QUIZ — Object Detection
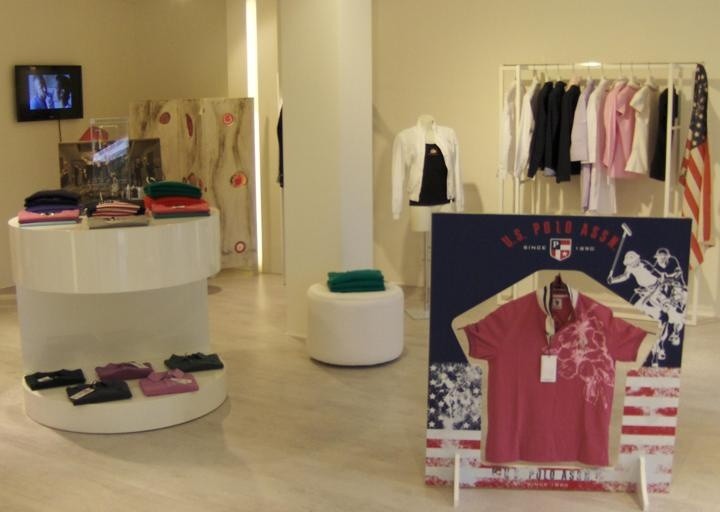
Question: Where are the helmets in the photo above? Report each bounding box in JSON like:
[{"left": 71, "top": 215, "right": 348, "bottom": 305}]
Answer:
[{"left": 623, "top": 251, "right": 640, "bottom": 266}]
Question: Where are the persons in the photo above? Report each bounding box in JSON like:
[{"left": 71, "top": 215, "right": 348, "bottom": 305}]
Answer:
[
  {"left": 648, "top": 248, "right": 686, "bottom": 347},
  {"left": 390, "top": 115, "right": 464, "bottom": 232},
  {"left": 29, "top": 76, "right": 52, "bottom": 109},
  {"left": 54, "top": 76, "right": 70, "bottom": 108},
  {"left": 608, "top": 251, "right": 670, "bottom": 363}
]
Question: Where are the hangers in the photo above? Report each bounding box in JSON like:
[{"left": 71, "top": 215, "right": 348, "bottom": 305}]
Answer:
[{"left": 547, "top": 62, "right": 663, "bottom": 94}]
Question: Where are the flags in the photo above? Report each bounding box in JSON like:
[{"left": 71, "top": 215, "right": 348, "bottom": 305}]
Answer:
[{"left": 679, "top": 65, "right": 713, "bottom": 269}]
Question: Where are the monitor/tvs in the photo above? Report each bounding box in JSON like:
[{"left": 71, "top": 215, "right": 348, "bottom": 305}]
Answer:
[{"left": 14, "top": 64, "right": 84, "bottom": 123}]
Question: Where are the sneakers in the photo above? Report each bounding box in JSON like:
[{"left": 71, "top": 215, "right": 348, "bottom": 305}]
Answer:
[{"left": 652, "top": 331, "right": 680, "bottom": 360}]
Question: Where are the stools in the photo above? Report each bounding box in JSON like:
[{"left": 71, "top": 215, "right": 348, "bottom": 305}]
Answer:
[{"left": 304, "top": 282, "right": 405, "bottom": 367}]
